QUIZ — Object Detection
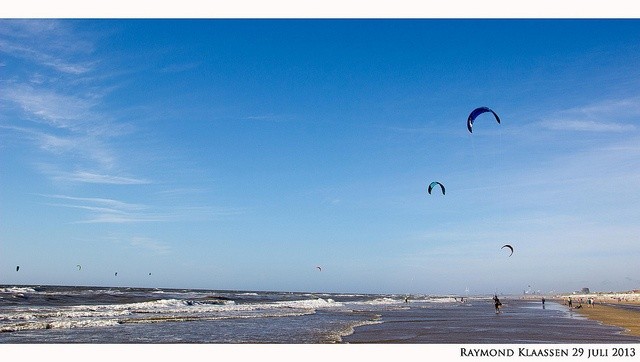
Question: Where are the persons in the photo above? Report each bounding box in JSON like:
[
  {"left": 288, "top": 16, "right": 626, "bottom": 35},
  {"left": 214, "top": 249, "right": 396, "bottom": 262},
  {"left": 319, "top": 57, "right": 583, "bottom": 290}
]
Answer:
[
  {"left": 493, "top": 296, "right": 500, "bottom": 314},
  {"left": 542, "top": 298, "right": 545, "bottom": 305},
  {"left": 567, "top": 297, "right": 595, "bottom": 309}
]
